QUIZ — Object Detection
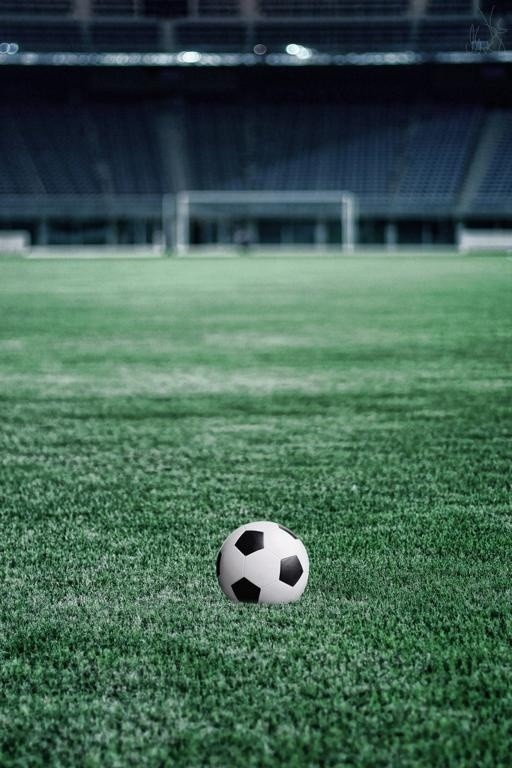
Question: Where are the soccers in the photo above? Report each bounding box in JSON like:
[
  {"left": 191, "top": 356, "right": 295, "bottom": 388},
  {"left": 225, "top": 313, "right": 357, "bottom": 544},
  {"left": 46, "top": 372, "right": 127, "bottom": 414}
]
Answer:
[{"left": 216, "top": 521, "right": 310, "bottom": 603}]
